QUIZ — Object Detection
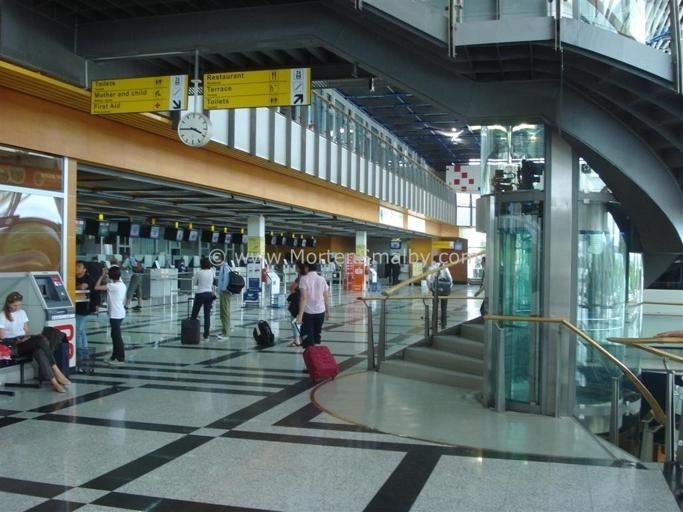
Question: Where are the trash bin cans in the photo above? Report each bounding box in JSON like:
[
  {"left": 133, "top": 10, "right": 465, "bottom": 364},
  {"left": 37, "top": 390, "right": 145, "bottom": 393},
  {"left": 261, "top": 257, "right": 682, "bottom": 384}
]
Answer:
[{"left": 76, "top": 347, "right": 96, "bottom": 375}]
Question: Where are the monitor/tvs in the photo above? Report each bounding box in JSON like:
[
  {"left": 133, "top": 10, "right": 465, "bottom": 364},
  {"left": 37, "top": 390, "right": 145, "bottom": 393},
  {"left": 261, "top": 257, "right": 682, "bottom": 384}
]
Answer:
[
  {"left": 87, "top": 221, "right": 110, "bottom": 238},
  {"left": 183, "top": 230, "right": 199, "bottom": 241},
  {"left": 141, "top": 225, "right": 159, "bottom": 239},
  {"left": 74, "top": 220, "right": 85, "bottom": 235},
  {"left": 118, "top": 222, "right": 140, "bottom": 238},
  {"left": 164, "top": 229, "right": 184, "bottom": 240},
  {"left": 37, "top": 284, "right": 47, "bottom": 297},
  {"left": 201, "top": 230, "right": 317, "bottom": 249}
]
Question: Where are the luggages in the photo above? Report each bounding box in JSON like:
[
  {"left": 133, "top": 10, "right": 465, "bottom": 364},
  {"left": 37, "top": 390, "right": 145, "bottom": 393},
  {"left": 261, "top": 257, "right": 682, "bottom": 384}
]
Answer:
[
  {"left": 181, "top": 298, "right": 200, "bottom": 344},
  {"left": 39, "top": 327, "right": 69, "bottom": 378},
  {"left": 295, "top": 322, "right": 338, "bottom": 379}
]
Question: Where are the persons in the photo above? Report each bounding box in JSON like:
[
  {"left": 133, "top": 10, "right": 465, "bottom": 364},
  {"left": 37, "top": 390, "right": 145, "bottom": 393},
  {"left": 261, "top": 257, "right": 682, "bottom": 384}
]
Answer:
[
  {"left": 191, "top": 257, "right": 215, "bottom": 342},
  {"left": 215, "top": 251, "right": 233, "bottom": 341},
  {"left": 474, "top": 278, "right": 488, "bottom": 314},
  {"left": 180, "top": 253, "right": 400, "bottom": 293},
  {"left": 473, "top": 257, "right": 486, "bottom": 315},
  {"left": 287, "top": 260, "right": 303, "bottom": 348},
  {"left": 74, "top": 259, "right": 93, "bottom": 374},
  {"left": 654, "top": 329, "right": 683, "bottom": 338},
  {"left": 427, "top": 255, "right": 453, "bottom": 330},
  {"left": 490, "top": 157, "right": 536, "bottom": 229},
  {"left": 116, "top": 253, "right": 144, "bottom": 311},
  {"left": 296, "top": 260, "right": 331, "bottom": 373},
  {"left": 93, "top": 265, "right": 129, "bottom": 364},
  {"left": 0, "top": 291, "right": 72, "bottom": 393}
]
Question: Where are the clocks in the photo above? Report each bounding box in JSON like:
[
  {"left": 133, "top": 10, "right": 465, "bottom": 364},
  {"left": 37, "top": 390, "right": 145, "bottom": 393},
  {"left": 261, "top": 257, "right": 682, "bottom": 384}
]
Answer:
[{"left": 177, "top": 112, "right": 212, "bottom": 147}]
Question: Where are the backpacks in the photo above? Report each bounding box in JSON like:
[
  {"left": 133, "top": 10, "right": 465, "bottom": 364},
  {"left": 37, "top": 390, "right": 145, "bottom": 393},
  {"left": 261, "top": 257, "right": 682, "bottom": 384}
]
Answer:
[
  {"left": 223, "top": 265, "right": 245, "bottom": 294},
  {"left": 253, "top": 320, "right": 274, "bottom": 345}
]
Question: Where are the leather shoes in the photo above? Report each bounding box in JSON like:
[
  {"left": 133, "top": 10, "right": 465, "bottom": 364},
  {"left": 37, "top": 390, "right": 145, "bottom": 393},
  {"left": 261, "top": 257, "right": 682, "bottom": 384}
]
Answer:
[{"left": 58, "top": 379, "right": 71, "bottom": 392}]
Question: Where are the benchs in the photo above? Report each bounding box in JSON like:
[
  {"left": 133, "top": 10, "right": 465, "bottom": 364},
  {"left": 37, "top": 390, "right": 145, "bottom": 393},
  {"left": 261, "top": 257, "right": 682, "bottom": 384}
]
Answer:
[{"left": 0, "top": 355, "right": 40, "bottom": 395}]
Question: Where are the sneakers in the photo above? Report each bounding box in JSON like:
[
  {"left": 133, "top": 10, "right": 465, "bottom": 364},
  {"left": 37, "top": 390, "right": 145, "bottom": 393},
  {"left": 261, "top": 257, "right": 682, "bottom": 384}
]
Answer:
[
  {"left": 104, "top": 358, "right": 124, "bottom": 364},
  {"left": 124, "top": 304, "right": 143, "bottom": 309}
]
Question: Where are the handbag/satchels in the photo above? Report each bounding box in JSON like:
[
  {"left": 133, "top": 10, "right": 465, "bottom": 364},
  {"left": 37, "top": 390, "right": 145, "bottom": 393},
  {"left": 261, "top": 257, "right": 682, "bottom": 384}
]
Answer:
[
  {"left": 132, "top": 261, "right": 144, "bottom": 273},
  {"left": 433, "top": 278, "right": 451, "bottom": 296}
]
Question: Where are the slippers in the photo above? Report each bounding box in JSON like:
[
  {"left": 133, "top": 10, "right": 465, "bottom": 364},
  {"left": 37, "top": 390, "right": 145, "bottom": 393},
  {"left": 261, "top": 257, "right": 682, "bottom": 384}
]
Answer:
[{"left": 288, "top": 342, "right": 301, "bottom": 346}]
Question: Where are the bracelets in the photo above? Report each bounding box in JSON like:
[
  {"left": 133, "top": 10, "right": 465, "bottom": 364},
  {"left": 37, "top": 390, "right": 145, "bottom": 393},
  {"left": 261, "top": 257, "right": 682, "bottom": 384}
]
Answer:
[{"left": 679, "top": 329, "right": 682, "bottom": 338}]
